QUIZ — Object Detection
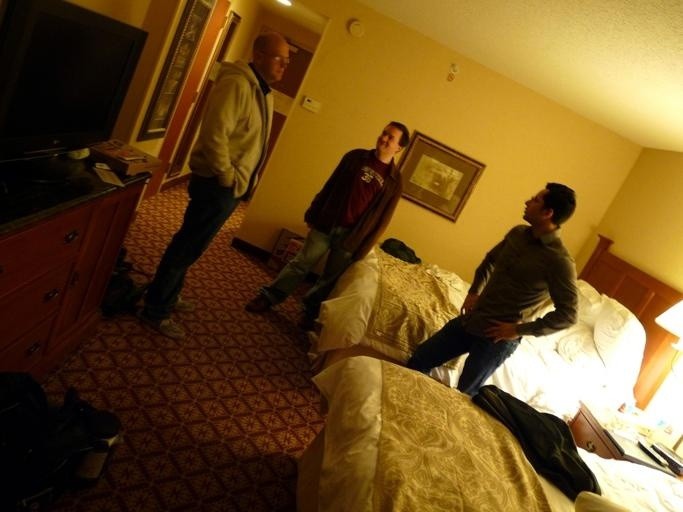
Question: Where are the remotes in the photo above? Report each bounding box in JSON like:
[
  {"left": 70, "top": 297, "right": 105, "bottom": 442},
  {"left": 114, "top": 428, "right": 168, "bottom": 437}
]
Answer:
[{"left": 639, "top": 438, "right": 669, "bottom": 467}]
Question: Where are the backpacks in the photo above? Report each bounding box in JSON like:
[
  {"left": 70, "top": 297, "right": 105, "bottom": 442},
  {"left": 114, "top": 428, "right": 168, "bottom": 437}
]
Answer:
[{"left": 0, "top": 370, "right": 82, "bottom": 512}]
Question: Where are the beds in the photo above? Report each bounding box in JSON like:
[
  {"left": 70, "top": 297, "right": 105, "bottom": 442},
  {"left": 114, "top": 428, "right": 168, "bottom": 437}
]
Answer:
[
  {"left": 294, "top": 356, "right": 683, "bottom": 512},
  {"left": 307, "top": 231, "right": 683, "bottom": 411}
]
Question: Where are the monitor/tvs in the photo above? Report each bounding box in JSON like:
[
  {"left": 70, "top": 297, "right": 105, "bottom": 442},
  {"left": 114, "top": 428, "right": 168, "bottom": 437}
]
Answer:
[{"left": 0, "top": 0, "right": 149, "bottom": 195}]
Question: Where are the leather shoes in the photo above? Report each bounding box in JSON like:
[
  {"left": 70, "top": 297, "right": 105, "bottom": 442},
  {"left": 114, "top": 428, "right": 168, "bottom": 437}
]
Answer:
[
  {"left": 245, "top": 294, "right": 271, "bottom": 312},
  {"left": 301, "top": 310, "right": 313, "bottom": 329}
]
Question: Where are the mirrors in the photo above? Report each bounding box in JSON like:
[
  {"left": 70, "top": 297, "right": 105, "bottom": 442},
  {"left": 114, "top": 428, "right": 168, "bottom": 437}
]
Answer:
[{"left": 167, "top": 10, "right": 242, "bottom": 178}]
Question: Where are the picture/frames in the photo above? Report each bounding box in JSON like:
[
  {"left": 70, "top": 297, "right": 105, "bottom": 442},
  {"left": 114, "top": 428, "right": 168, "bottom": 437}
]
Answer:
[
  {"left": 135, "top": 0, "right": 220, "bottom": 143},
  {"left": 398, "top": 131, "right": 485, "bottom": 223}
]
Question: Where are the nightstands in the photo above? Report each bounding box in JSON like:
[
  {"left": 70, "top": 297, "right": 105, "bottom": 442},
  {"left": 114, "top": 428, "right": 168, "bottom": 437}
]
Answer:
[{"left": 567, "top": 400, "right": 682, "bottom": 482}]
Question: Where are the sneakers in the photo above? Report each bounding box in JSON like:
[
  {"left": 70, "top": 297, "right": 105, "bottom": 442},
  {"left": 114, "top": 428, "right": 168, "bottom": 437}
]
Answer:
[
  {"left": 136, "top": 308, "right": 186, "bottom": 340},
  {"left": 141, "top": 287, "right": 196, "bottom": 312}
]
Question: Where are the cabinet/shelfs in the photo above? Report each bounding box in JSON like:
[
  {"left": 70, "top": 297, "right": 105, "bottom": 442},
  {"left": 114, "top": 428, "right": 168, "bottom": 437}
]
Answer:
[{"left": 1, "top": 177, "right": 147, "bottom": 386}]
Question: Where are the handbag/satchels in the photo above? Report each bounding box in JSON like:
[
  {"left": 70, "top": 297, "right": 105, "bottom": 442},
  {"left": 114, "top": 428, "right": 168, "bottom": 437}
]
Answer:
[
  {"left": 380, "top": 238, "right": 422, "bottom": 264},
  {"left": 53, "top": 385, "right": 123, "bottom": 493},
  {"left": 99, "top": 244, "right": 155, "bottom": 317}
]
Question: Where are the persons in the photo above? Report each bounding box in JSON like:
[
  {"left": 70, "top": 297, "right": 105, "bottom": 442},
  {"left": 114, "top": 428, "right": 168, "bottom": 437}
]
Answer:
[
  {"left": 134, "top": 29, "right": 291, "bottom": 341},
  {"left": 243, "top": 119, "right": 411, "bottom": 334},
  {"left": 400, "top": 181, "right": 582, "bottom": 397}
]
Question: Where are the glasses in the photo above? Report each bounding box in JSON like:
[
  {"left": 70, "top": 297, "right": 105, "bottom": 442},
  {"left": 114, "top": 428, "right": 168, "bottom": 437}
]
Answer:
[{"left": 259, "top": 49, "right": 291, "bottom": 65}]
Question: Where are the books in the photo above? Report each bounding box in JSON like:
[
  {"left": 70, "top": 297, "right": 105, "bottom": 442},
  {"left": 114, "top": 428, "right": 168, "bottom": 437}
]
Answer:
[{"left": 603, "top": 427, "right": 683, "bottom": 478}]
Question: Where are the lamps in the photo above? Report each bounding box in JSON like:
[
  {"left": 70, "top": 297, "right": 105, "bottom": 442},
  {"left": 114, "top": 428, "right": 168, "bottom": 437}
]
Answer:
[{"left": 651, "top": 297, "right": 683, "bottom": 474}]
